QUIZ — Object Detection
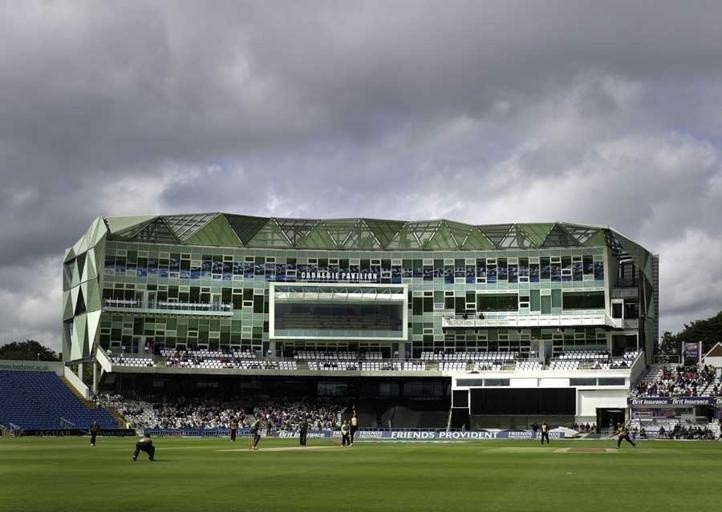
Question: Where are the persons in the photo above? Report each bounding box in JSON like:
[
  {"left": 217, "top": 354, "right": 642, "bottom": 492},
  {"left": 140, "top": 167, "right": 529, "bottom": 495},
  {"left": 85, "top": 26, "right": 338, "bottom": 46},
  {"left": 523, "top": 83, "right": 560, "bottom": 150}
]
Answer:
[
  {"left": 533, "top": 365, "right": 721, "bottom": 448},
  {"left": 91, "top": 392, "right": 361, "bottom": 462},
  {"left": 346, "top": 364, "right": 397, "bottom": 371}
]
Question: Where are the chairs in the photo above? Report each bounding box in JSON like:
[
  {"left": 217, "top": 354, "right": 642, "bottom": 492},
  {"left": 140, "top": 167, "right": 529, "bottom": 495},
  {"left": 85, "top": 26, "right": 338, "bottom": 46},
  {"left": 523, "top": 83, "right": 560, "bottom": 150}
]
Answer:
[{"left": 108, "top": 348, "right": 638, "bottom": 372}]
